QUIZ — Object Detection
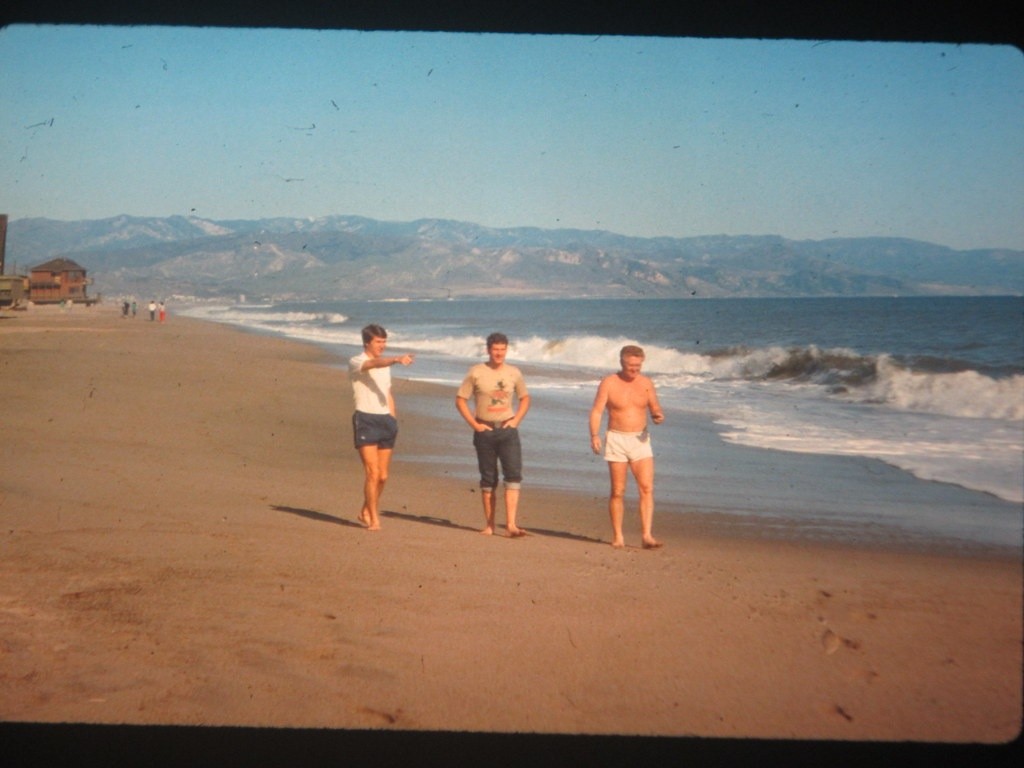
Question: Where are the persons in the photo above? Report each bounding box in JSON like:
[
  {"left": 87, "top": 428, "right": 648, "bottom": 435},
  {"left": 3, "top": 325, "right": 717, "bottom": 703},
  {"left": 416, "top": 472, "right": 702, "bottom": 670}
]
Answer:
[
  {"left": 122, "top": 300, "right": 166, "bottom": 323},
  {"left": 589, "top": 344, "right": 666, "bottom": 549},
  {"left": 348, "top": 323, "right": 415, "bottom": 530},
  {"left": 455, "top": 332, "right": 531, "bottom": 538},
  {"left": 59, "top": 297, "right": 73, "bottom": 312}
]
